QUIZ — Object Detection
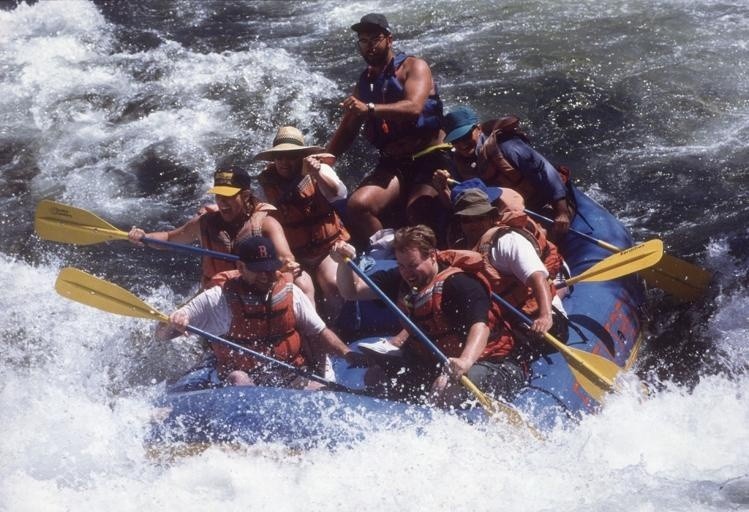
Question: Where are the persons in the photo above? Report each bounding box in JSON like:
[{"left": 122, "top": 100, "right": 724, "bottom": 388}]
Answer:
[
  {"left": 130, "top": 106, "right": 568, "bottom": 408},
  {"left": 317, "top": 13, "right": 456, "bottom": 236}
]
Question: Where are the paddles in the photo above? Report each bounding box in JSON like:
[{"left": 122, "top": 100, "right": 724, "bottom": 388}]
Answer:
[
  {"left": 33, "top": 196, "right": 292, "bottom": 272},
  {"left": 54, "top": 266, "right": 378, "bottom": 400},
  {"left": 442, "top": 177, "right": 715, "bottom": 301},
  {"left": 437, "top": 257, "right": 622, "bottom": 407},
  {"left": 553, "top": 238, "right": 664, "bottom": 290},
  {"left": 342, "top": 257, "right": 547, "bottom": 447}
]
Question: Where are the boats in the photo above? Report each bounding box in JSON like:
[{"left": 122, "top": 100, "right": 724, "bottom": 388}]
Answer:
[{"left": 149, "top": 187, "right": 642, "bottom": 465}]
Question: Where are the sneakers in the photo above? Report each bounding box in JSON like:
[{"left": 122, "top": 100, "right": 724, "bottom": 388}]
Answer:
[{"left": 357, "top": 338, "right": 403, "bottom": 361}]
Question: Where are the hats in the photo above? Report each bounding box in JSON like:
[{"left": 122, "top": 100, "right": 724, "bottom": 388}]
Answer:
[{"left": 351, "top": 12, "right": 391, "bottom": 34}]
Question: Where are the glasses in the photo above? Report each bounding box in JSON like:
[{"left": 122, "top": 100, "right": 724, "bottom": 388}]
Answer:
[{"left": 357, "top": 35, "right": 384, "bottom": 47}]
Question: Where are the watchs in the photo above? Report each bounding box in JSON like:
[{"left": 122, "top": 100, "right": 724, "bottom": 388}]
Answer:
[{"left": 365, "top": 101, "right": 377, "bottom": 116}]
